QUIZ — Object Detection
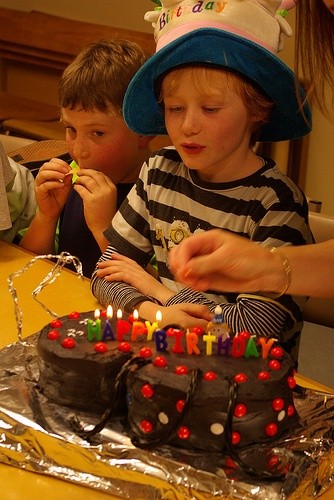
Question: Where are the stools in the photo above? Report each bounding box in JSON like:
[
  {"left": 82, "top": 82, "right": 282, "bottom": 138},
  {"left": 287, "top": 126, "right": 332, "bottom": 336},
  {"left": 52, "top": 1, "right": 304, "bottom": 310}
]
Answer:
[
  {"left": 0, "top": 92, "right": 63, "bottom": 121},
  {"left": 0, "top": 118, "right": 66, "bottom": 140}
]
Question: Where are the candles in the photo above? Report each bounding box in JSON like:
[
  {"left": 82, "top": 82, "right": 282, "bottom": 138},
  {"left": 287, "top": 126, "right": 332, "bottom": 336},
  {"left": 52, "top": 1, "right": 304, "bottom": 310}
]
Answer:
[{"left": 87, "top": 304, "right": 278, "bottom": 359}]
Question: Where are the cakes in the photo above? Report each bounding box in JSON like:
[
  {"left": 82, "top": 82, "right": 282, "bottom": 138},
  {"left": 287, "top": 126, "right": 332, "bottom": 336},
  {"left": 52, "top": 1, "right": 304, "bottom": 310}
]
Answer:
[
  {"left": 36, "top": 308, "right": 161, "bottom": 415},
  {"left": 126, "top": 326, "right": 302, "bottom": 454}
]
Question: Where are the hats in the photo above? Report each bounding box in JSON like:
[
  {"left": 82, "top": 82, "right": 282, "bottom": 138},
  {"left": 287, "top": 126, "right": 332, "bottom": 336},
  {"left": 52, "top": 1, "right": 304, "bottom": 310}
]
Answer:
[{"left": 123, "top": 0, "right": 313, "bottom": 142}]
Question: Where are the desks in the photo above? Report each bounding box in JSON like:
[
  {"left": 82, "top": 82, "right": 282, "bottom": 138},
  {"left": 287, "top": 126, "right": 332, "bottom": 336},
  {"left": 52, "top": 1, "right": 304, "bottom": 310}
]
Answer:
[{"left": 0, "top": 235, "right": 334, "bottom": 500}]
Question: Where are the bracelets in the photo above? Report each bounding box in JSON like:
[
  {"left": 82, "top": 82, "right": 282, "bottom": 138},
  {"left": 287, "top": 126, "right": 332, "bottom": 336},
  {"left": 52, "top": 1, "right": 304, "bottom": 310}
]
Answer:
[{"left": 263, "top": 246, "right": 291, "bottom": 298}]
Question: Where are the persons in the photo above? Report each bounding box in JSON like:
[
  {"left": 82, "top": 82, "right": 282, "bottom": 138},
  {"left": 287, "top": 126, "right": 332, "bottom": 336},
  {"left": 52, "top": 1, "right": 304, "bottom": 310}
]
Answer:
[
  {"left": 19, "top": 39, "right": 157, "bottom": 282},
  {"left": 167, "top": 0, "right": 334, "bottom": 301},
  {"left": 0, "top": 139, "right": 39, "bottom": 243},
  {"left": 88, "top": 0, "right": 314, "bottom": 371}
]
